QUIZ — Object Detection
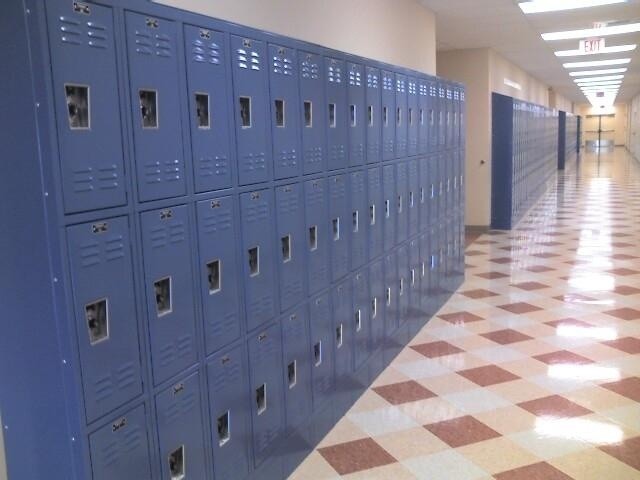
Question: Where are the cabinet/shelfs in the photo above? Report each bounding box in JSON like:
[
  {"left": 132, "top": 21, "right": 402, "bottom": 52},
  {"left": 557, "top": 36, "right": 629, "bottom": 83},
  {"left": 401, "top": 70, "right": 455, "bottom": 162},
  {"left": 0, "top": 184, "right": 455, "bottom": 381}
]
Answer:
[{"left": 491, "top": 91, "right": 584, "bottom": 230}]
